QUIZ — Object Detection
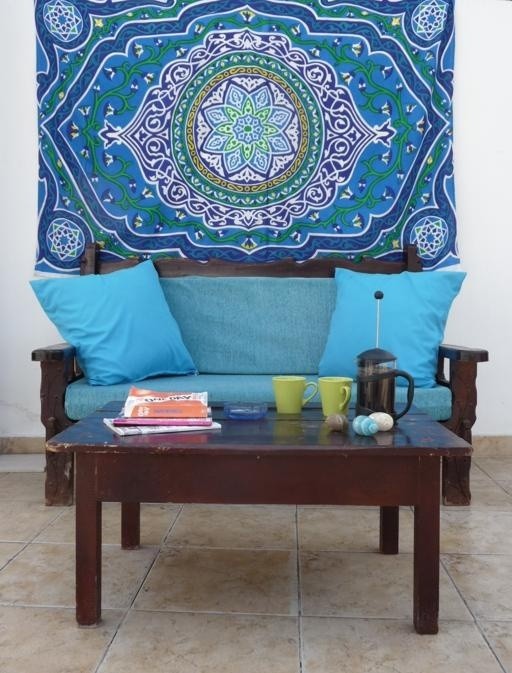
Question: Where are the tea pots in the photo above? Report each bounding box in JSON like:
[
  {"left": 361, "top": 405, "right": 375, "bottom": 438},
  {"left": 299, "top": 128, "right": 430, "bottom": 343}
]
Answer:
[{"left": 356, "top": 291, "right": 414, "bottom": 426}]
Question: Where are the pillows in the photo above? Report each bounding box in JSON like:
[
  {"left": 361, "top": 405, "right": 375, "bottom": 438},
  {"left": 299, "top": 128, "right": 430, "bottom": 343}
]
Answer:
[
  {"left": 318, "top": 266, "right": 467, "bottom": 389},
  {"left": 29, "top": 258, "right": 200, "bottom": 388}
]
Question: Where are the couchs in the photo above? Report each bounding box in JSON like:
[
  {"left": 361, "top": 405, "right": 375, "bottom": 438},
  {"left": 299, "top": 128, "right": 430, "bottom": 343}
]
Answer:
[{"left": 31, "top": 241, "right": 490, "bottom": 509}]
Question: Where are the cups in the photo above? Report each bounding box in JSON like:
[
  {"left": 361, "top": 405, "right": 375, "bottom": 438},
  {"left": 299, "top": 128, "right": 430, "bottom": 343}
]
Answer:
[{"left": 271, "top": 376, "right": 353, "bottom": 417}]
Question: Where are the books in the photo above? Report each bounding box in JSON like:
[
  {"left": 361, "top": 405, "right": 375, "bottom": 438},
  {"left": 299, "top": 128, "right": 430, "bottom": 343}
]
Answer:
[
  {"left": 99, "top": 417, "right": 224, "bottom": 438},
  {"left": 109, "top": 414, "right": 214, "bottom": 428},
  {"left": 122, "top": 384, "right": 210, "bottom": 419}
]
